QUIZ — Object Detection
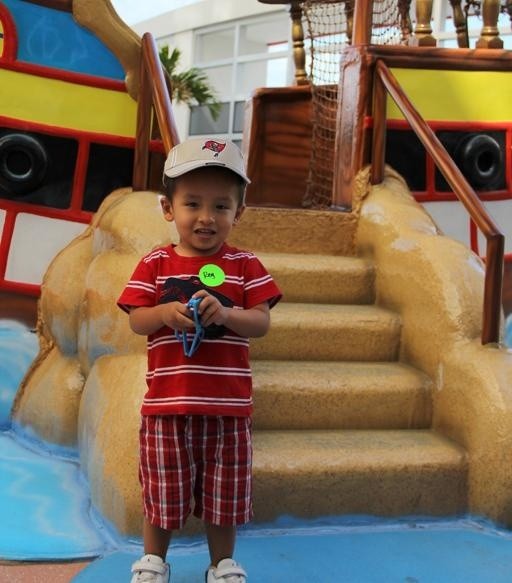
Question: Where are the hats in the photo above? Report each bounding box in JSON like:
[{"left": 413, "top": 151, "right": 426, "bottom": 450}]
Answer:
[{"left": 163, "top": 137, "right": 252, "bottom": 186}]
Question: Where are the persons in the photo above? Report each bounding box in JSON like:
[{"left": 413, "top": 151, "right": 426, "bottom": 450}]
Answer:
[{"left": 118, "top": 135, "right": 282, "bottom": 583}]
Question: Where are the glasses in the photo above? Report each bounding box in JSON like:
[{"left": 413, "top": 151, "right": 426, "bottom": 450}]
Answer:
[{"left": 176, "top": 298, "right": 205, "bottom": 358}]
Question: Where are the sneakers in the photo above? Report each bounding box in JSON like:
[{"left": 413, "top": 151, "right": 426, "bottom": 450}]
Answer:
[
  {"left": 130, "top": 553, "right": 169, "bottom": 583},
  {"left": 205, "top": 558, "right": 248, "bottom": 583}
]
